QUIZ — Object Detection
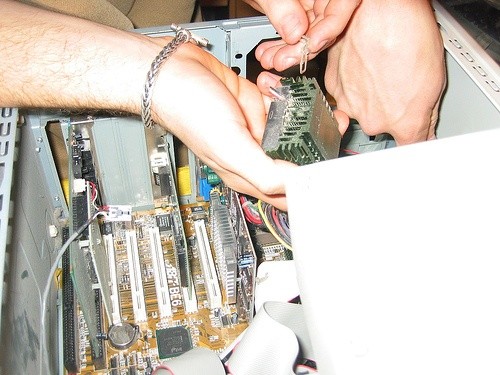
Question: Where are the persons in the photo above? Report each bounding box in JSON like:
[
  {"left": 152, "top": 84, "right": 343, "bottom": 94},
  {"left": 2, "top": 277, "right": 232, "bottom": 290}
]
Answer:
[
  {"left": 0, "top": 0, "right": 364, "bottom": 212},
  {"left": 325, "top": 0, "right": 447, "bottom": 147}
]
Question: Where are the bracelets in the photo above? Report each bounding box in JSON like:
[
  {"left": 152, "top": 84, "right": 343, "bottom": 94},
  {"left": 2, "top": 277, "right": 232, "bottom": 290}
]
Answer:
[{"left": 142, "top": 25, "right": 208, "bottom": 129}]
